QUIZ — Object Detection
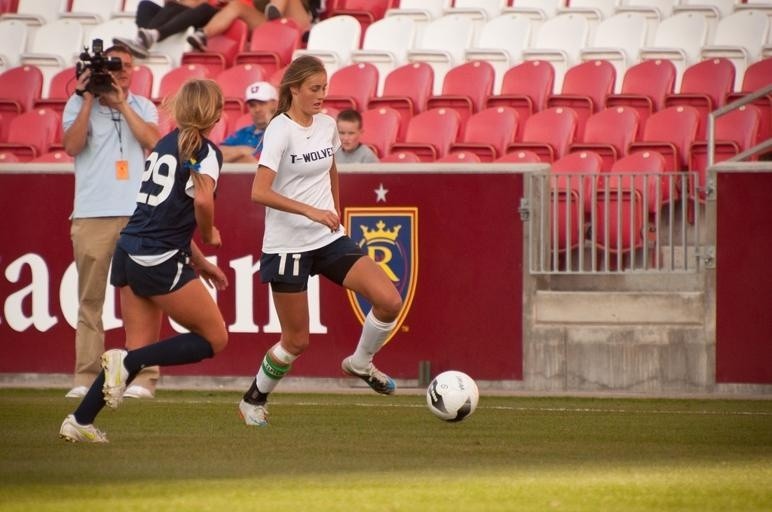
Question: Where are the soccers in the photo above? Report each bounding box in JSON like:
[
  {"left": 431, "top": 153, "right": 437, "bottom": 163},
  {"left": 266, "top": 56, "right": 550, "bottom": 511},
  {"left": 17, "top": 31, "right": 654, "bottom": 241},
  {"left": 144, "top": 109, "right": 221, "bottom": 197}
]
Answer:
[{"left": 424, "top": 368, "right": 481, "bottom": 424}]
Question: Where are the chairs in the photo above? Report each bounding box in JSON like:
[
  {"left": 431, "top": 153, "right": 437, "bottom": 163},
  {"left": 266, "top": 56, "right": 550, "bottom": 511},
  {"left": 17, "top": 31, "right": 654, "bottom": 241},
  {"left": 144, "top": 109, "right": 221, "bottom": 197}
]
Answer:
[
  {"left": 152, "top": 64, "right": 208, "bottom": 110},
  {"left": 2, "top": 1, "right": 70, "bottom": 25},
  {"left": 484, "top": 59, "right": 553, "bottom": 140},
  {"left": 426, "top": 60, "right": 494, "bottom": 137},
  {"left": 0, "top": 64, "right": 43, "bottom": 113},
  {"left": 581, "top": 11, "right": 655, "bottom": 95},
  {"left": 546, "top": 59, "right": 616, "bottom": 143},
  {"left": 170, "top": 110, "right": 226, "bottom": 144},
  {"left": 136, "top": 31, "right": 192, "bottom": 100},
  {"left": 432, "top": 152, "right": 480, "bottom": 163},
  {"left": 466, "top": 14, "right": 532, "bottom": 95},
  {"left": 181, "top": 21, "right": 247, "bottom": 77},
  {"left": 111, "top": 0, "right": 164, "bottom": 20},
  {"left": 384, "top": 0, "right": 452, "bottom": 29},
  {"left": 60, "top": 1, "right": 123, "bottom": 20},
  {"left": 556, "top": 0, "right": 622, "bottom": 24},
  {"left": 592, "top": 151, "right": 665, "bottom": 271},
  {"left": 19, "top": 19, "right": 84, "bottom": 98},
  {"left": 449, "top": 104, "right": 518, "bottom": 163},
  {"left": 699, "top": 8, "right": 771, "bottom": 92},
  {"left": 291, "top": 15, "right": 362, "bottom": 89},
  {"left": 0, "top": 107, "right": 59, "bottom": 164},
  {"left": 667, "top": 0, "right": 700, "bottom": 13},
  {"left": 445, "top": 0, "right": 507, "bottom": 23},
  {"left": 389, "top": 108, "right": 461, "bottom": 161},
  {"left": 630, "top": 104, "right": 701, "bottom": 204},
  {"left": 368, "top": 62, "right": 432, "bottom": 143},
  {"left": 640, "top": 10, "right": 716, "bottom": 93},
  {"left": 352, "top": 14, "right": 419, "bottom": 98},
  {"left": 129, "top": 65, "right": 152, "bottom": 101},
  {"left": 507, "top": 106, "right": 578, "bottom": 165},
  {"left": 357, "top": 106, "right": 401, "bottom": 157},
  {"left": 604, "top": 58, "right": 676, "bottom": 142},
  {"left": 408, "top": 13, "right": 475, "bottom": 95},
  {"left": 726, "top": 57, "right": 772, "bottom": 145},
  {"left": 568, "top": 105, "right": 640, "bottom": 187},
  {"left": 234, "top": 19, "right": 303, "bottom": 83},
  {"left": 379, "top": 153, "right": 421, "bottom": 163},
  {"left": 73, "top": 19, "right": 139, "bottom": 61},
  {"left": 214, "top": 63, "right": 265, "bottom": 113},
  {"left": 687, "top": 103, "right": 762, "bottom": 224},
  {"left": 501, "top": 0, "right": 567, "bottom": 23},
  {"left": 36, "top": 65, "right": 78, "bottom": 115},
  {"left": 489, "top": 151, "right": 542, "bottom": 165},
  {"left": 0, "top": 16, "right": 36, "bottom": 72},
  {"left": 549, "top": 149, "right": 601, "bottom": 259},
  {"left": 321, "top": 62, "right": 378, "bottom": 129},
  {"left": 664, "top": 57, "right": 735, "bottom": 142},
  {"left": 614, "top": 0, "right": 677, "bottom": 23},
  {"left": 233, "top": 113, "right": 255, "bottom": 132},
  {"left": 522, "top": 12, "right": 591, "bottom": 94}
]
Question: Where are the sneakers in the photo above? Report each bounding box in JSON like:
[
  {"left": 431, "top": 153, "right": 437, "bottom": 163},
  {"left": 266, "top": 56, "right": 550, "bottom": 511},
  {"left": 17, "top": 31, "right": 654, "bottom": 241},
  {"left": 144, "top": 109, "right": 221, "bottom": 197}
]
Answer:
[
  {"left": 237, "top": 377, "right": 270, "bottom": 426},
  {"left": 59, "top": 413, "right": 112, "bottom": 444},
  {"left": 266, "top": 4, "right": 280, "bottom": 20},
  {"left": 342, "top": 354, "right": 395, "bottom": 395},
  {"left": 99, "top": 348, "right": 129, "bottom": 411},
  {"left": 187, "top": 27, "right": 208, "bottom": 51},
  {"left": 114, "top": 38, "right": 150, "bottom": 59},
  {"left": 137, "top": 28, "right": 154, "bottom": 49},
  {"left": 64, "top": 386, "right": 88, "bottom": 398},
  {"left": 124, "top": 384, "right": 154, "bottom": 399}
]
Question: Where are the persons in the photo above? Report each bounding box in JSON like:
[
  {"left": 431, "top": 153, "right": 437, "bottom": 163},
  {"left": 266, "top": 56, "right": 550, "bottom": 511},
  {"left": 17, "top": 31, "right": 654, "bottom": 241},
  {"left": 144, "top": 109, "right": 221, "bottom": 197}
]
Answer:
[
  {"left": 57, "top": 78, "right": 229, "bottom": 445},
  {"left": 217, "top": 81, "right": 280, "bottom": 163},
  {"left": 334, "top": 108, "right": 379, "bottom": 162},
  {"left": 238, "top": 55, "right": 402, "bottom": 428},
  {"left": 187, "top": 0, "right": 324, "bottom": 52},
  {"left": 61, "top": 45, "right": 161, "bottom": 398},
  {"left": 112, "top": 0, "right": 221, "bottom": 58}
]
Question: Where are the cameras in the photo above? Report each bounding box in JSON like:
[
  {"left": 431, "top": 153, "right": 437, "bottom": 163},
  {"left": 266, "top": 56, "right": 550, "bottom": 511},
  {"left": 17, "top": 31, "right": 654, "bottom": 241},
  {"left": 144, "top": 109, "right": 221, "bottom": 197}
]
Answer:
[{"left": 77, "top": 39, "right": 121, "bottom": 97}]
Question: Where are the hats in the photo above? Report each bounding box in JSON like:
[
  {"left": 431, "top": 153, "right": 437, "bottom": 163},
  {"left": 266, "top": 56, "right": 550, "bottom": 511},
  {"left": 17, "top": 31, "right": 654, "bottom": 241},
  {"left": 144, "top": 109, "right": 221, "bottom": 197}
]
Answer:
[{"left": 245, "top": 81, "right": 280, "bottom": 101}]
